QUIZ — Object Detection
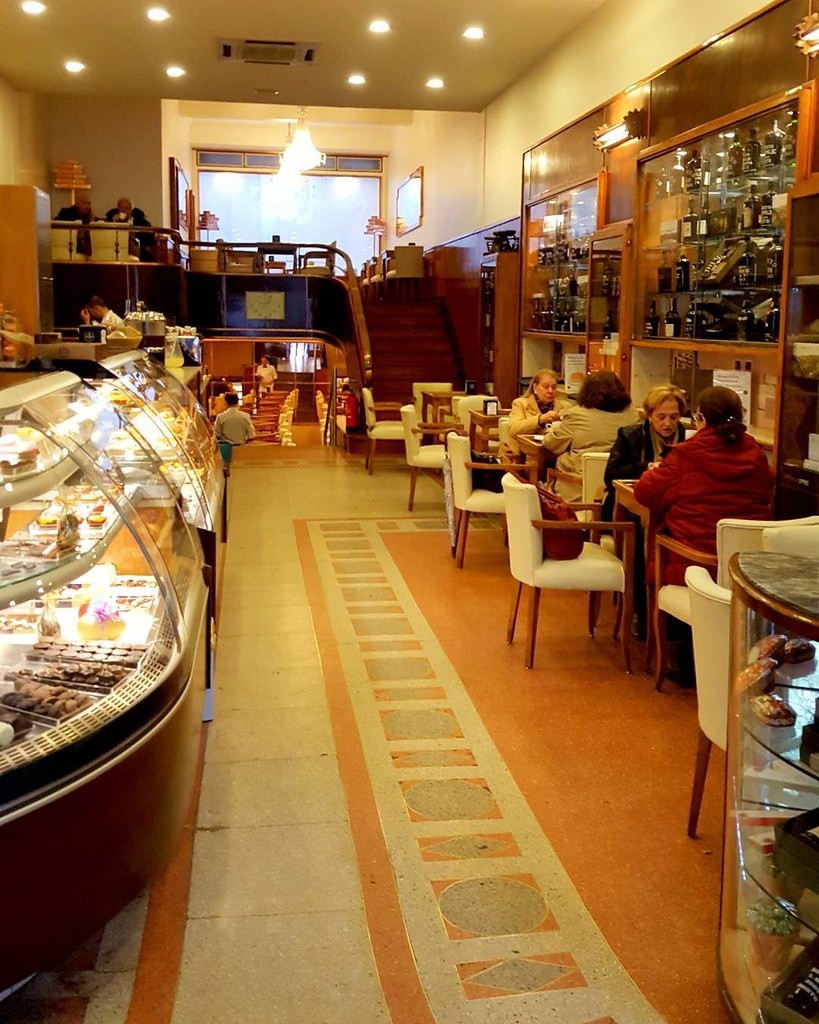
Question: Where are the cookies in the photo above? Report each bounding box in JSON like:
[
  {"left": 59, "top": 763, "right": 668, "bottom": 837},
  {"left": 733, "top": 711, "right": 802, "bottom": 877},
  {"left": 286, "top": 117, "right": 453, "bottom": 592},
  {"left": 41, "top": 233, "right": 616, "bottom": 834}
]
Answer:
[
  {"left": 1, "top": 428, "right": 160, "bottom": 632},
  {"left": 0, "top": 637, "right": 147, "bottom": 734},
  {"left": 112, "top": 375, "right": 204, "bottom": 502}
]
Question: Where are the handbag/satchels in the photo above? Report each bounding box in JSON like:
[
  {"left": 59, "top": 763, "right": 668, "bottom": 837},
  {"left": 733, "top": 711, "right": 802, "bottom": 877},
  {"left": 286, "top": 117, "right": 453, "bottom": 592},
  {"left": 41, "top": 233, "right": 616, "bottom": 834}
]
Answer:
[
  {"left": 471, "top": 449, "right": 506, "bottom": 493},
  {"left": 523, "top": 480, "right": 583, "bottom": 561}
]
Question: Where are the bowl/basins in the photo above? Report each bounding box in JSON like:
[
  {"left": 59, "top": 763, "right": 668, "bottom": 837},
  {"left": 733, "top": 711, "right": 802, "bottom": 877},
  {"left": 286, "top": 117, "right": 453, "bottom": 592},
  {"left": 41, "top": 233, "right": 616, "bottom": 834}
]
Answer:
[
  {"left": 107, "top": 337, "right": 143, "bottom": 349},
  {"left": 124, "top": 319, "right": 167, "bottom": 335}
]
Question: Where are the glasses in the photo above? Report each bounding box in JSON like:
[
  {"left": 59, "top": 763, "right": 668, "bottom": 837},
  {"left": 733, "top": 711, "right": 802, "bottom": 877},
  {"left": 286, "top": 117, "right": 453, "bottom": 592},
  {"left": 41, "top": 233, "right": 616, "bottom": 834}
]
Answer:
[{"left": 691, "top": 413, "right": 703, "bottom": 422}]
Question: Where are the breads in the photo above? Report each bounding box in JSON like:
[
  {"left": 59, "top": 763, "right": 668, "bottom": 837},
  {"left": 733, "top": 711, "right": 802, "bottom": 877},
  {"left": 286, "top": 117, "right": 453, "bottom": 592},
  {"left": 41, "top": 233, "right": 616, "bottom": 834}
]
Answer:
[
  {"left": 748, "top": 694, "right": 798, "bottom": 725},
  {"left": 780, "top": 639, "right": 815, "bottom": 664}
]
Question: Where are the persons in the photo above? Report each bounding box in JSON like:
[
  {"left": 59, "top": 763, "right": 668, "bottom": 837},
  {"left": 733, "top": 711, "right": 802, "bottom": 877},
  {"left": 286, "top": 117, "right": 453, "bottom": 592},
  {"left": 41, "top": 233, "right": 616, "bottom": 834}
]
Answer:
[
  {"left": 634, "top": 387, "right": 776, "bottom": 687},
  {"left": 543, "top": 370, "right": 642, "bottom": 505},
  {"left": 602, "top": 384, "right": 685, "bottom": 640},
  {"left": 507, "top": 369, "right": 571, "bottom": 467},
  {"left": 213, "top": 390, "right": 256, "bottom": 445},
  {"left": 105, "top": 197, "right": 158, "bottom": 262},
  {"left": 80, "top": 293, "right": 123, "bottom": 330},
  {"left": 49, "top": 189, "right": 97, "bottom": 257},
  {"left": 256, "top": 356, "right": 279, "bottom": 392}
]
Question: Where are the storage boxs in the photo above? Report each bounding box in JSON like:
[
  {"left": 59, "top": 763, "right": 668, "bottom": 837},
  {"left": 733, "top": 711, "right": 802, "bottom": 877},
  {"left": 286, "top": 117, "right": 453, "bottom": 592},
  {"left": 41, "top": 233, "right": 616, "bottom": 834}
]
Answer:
[{"left": 32, "top": 343, "right": 107, "bottom": 363}]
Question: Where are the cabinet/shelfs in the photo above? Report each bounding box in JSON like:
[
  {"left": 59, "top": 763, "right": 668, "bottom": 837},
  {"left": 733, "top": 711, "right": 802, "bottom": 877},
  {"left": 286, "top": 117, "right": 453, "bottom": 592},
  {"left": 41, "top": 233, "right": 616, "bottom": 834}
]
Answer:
[
  {"left": 0, "top": 349, "right": 230, "bottom": 999},
  {"left": 718, "top": 551, "right": 819, "bottom": 1024},
  {"left": 479, "top": 0, "right": 819, "bottom": 500}
]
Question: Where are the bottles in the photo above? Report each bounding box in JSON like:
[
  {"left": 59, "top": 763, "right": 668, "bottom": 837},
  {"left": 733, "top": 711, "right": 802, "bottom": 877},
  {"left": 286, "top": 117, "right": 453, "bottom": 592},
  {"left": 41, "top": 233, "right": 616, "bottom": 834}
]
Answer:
[
  {"left": 644, "top": 111, "right": 799, "bottom": 342},
  {"left": 532, "top": 243, "right": 614, "bottom": 333}
]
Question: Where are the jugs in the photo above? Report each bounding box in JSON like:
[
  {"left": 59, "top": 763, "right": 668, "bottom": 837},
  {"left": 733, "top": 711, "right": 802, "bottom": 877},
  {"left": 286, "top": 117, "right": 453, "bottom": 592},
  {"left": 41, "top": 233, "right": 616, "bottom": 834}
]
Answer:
[{"left": 165, "top": 334, "right": 184, "bottom": 369}]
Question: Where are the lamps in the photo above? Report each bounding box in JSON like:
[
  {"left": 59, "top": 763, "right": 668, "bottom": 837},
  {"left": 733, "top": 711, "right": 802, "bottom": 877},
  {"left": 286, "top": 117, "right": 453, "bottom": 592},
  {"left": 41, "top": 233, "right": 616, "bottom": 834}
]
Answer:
[
  {"left": 592, "top": 108, "right": 641, "bottom": 154},
  {"left": 794, "top": 12, "right": 819, "bottom": 58}
]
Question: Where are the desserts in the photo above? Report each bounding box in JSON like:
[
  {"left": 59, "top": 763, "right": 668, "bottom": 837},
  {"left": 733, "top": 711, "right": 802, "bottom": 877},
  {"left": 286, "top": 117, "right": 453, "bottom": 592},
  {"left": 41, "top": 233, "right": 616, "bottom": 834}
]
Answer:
[{"left": 734, "top": 633, "right": 788, "bottom": 702}]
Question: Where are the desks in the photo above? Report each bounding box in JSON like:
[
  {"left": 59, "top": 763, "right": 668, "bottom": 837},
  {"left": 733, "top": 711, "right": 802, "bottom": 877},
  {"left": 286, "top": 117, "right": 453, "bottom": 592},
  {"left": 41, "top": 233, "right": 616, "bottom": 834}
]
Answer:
[
  {"left": 382, "top": 250, "right": 393, "bottom": 301},
  {"left": 612, "top": 477, "right": 659, "bottom": 671},
  {"left": 468, "top": 409, "right": 511, "bottom": 462},
  {"left": 257, "top": 243, "right": 296, "bottom": 275},
  {"left": 516, "top": 433, "right": 557, "bottom": 483},
  {"left": 421, "top": 391, "right": 486, "bottom": 476}
]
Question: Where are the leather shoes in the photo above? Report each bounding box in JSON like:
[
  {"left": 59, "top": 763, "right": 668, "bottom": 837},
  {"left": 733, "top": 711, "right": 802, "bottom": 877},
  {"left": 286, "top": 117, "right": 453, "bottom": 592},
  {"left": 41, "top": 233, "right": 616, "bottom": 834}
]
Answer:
[{"left": 630, "top": 614, "right": 640, "bottom": 640}]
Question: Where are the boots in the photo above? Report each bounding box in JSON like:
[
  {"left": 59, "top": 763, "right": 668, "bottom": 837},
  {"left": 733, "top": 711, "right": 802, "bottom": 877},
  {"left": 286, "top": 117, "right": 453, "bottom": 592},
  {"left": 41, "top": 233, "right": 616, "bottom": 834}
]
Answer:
[{"left": 665, "top": 640, "right": 680, "bottom": 678}]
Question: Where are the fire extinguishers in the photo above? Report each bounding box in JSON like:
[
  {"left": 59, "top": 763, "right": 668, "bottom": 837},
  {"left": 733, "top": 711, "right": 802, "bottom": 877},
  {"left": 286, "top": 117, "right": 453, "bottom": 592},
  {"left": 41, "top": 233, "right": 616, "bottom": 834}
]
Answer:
[{"left": 342, "top": 384, "right": 359, "bottom": 427}]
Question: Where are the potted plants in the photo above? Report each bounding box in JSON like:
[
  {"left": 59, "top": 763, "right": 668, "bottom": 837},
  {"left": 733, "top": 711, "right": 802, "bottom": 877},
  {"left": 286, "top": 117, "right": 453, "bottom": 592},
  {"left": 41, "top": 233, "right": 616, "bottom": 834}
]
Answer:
[{"left": 746, "top": 897, "right": 803, "bottom": 970}]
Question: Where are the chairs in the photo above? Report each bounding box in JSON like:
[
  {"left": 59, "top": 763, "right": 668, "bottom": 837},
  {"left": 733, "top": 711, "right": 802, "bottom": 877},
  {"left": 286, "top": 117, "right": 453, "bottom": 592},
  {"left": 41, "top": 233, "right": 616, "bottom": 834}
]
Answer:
[
  {"left": 189, "top": 240, "right": 336, "bottom": 277},
  {"left": 211, "top": 388, "right": 298, "bottom": 447},
  {"left": 50, "top": 220, "right": 86, "bottom": 261},
  {"left": 89, "top": 222, "right": 140, "bottom": 262},
  {"left": 358, "top": 244, "right": 426, "bottom": 303},
  {"left": 316, "top": 390, "right": 343, "bottom": 445},
  {"left": 362, "top": 383, "right": 819, "bottom": 839}
]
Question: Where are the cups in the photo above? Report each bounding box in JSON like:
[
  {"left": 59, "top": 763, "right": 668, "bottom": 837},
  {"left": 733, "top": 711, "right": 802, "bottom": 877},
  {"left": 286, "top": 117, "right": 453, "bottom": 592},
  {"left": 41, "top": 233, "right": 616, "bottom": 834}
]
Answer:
[
  {"left": 486, "top": 383, "right": 493, "bottom": 395},
  {"left": 272, "top": 235, "right": 280, "bottom": 243},
  {"left": 118, "top": 212, "right": 126, "bottom": 220},
  {"left": 545, "top": 421, "right": 562, "bottom": 429},
  {"left": 269, "top": 256, "right": 274, "bottom": 262},
  {"left": 464, "top": 380, "right": 477, "bottom": 396}
]
